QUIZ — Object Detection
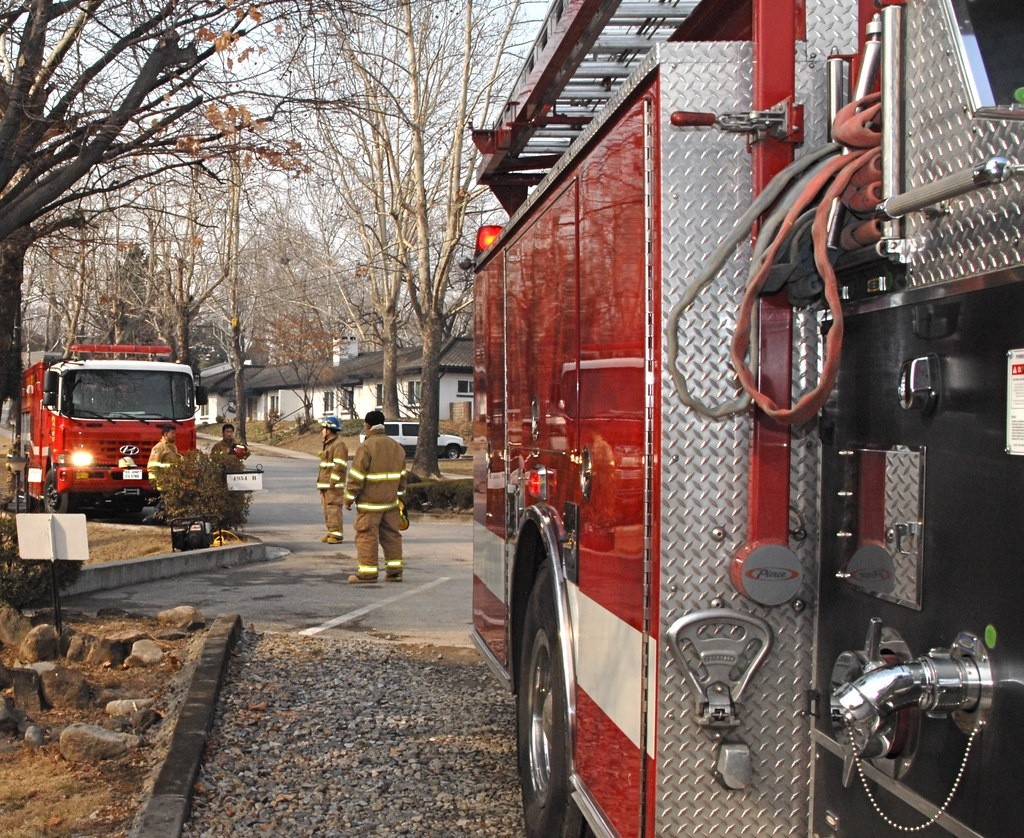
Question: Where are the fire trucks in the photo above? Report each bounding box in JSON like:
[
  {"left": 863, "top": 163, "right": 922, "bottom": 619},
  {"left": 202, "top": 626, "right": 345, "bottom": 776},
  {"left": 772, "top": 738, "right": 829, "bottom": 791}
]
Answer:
[
  {"left": 467, "top": 0, "right": 1024, "bottom": 836},
  {"left": 19, "top": 343, "right": 209, "bottom": 514}
]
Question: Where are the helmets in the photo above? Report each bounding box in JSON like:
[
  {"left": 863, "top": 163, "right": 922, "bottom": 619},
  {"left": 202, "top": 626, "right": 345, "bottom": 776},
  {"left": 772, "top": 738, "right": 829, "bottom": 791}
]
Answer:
[
  {"left": 319, "top": 417, "right": 342, "bottom": 429},
  {"left": 233, "top": 446, "right": 246, "bottom": 459}
]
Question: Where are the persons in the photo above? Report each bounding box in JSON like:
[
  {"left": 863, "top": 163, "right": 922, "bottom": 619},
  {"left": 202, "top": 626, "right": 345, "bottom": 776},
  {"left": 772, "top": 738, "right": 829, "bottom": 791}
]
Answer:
[
  {"left": 320, "top": 417, "right": 348, "bottom": 544},
  {"left": 343, "top": 411, "right": 406, "bottom": 583},
  {"left": 209, "top": 424, "right": 252, "bottom": 467},
  {"left": 2, "top": 433, "right": 21, "bottom": 503},
  {"left": 147, "top": 425, "right": 185, "bottom": 496}
]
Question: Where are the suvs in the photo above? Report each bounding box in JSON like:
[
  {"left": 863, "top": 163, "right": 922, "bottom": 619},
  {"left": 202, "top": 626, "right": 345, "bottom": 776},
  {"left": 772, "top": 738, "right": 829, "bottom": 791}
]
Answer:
[{"left": 359, "top": 421, "right": 468, "bottom": 461}]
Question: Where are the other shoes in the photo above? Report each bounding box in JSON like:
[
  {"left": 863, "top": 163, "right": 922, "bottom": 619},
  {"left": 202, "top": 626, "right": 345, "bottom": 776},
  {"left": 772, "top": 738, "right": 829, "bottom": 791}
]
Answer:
[
  {"left": 348, "top": 574, "right": 377, "bottom": 583},
  {"left": 327, "top": 538, "right": 343, "bottom": 543},
  {"left": 384, "top": 575, "right": 402, "bottom": 582},
  {"left": 320, "top": 536, "right": 328, "bottom": 542}
]
